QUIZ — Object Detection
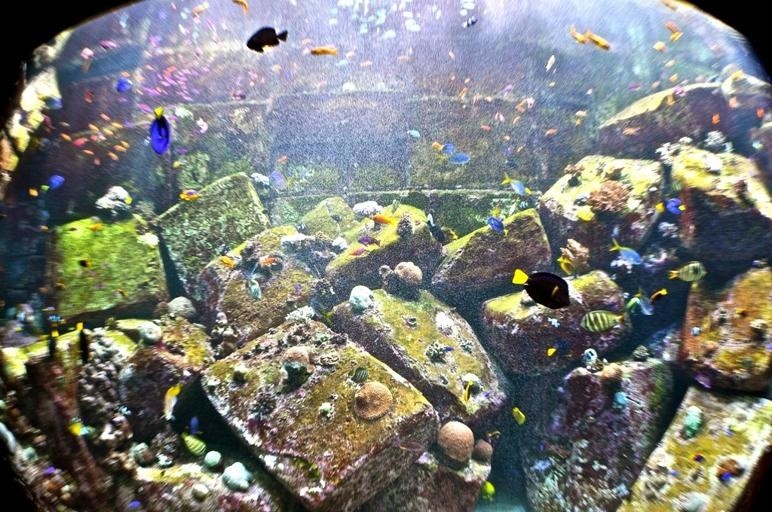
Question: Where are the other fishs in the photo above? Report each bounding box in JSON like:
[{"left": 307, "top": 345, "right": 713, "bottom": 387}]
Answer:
[{"left": 0, "top": 1, "right": 771, "bottom": 512}]
